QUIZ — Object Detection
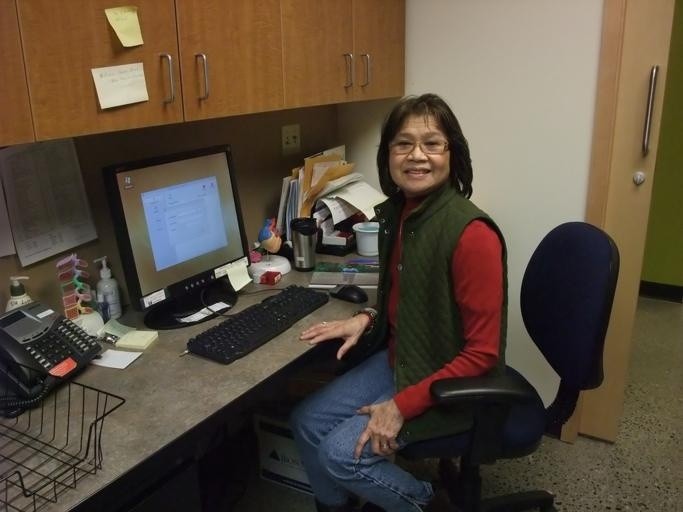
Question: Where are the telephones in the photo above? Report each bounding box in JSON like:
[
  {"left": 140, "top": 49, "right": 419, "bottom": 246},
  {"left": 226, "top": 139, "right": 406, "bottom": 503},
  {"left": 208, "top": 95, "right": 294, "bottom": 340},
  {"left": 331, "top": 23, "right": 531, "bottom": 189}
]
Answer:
[{"left": 0, "top": 302, "right": 102, "bottom": 404}]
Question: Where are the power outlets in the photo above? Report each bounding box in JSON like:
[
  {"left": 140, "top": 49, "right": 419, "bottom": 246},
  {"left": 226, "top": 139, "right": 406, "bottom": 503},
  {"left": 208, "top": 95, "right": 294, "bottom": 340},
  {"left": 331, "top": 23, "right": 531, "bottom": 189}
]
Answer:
[{"left": 281, "top": 124, "right": 302, "bottom": 155}]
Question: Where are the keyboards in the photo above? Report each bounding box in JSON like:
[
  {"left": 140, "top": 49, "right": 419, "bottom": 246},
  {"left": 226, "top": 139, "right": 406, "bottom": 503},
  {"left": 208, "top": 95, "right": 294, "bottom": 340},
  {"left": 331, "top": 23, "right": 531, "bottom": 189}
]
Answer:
[{"left": 186, "top": 284, "right": 330, "bottom": 366}]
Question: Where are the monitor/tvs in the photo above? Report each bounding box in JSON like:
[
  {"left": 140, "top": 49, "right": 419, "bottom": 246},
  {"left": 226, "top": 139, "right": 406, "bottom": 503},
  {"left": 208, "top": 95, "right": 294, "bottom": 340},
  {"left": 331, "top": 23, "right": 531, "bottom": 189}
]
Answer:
[{"left": 100, "top": 142, "right": 251, "bottom": 331}]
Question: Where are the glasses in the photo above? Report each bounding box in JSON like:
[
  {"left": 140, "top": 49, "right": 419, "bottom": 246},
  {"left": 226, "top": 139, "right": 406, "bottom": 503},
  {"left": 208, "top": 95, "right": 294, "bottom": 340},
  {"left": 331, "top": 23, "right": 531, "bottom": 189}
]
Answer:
[{"left": 389, "top": 138, "right": 448, "bottom": 153}]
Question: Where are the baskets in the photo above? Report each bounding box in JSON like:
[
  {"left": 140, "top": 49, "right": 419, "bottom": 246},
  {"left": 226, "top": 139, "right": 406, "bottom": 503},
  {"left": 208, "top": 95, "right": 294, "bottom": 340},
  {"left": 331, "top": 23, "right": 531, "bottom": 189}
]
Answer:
[{"left": 0, "top": 362, "right": 125, "bottom": 512}]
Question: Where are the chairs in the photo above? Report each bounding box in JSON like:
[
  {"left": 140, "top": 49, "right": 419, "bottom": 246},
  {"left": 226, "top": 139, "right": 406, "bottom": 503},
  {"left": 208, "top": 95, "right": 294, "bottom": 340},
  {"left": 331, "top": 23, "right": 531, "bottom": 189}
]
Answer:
[{"left": 402, "top": 222, "right": 621, "bottom": 512}]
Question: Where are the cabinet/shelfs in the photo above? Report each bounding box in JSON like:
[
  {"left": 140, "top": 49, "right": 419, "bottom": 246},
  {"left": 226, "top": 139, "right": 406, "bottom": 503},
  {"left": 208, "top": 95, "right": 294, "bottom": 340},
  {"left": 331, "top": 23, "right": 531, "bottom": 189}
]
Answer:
[{"left": 0, "top": 0, "right": 406, "bottom": 148}]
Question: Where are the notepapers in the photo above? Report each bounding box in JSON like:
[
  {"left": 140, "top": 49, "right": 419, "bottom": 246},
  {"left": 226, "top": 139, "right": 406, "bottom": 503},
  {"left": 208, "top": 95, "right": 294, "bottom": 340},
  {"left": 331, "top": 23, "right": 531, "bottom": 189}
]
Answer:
[{"left": 115, "top": 330, "right": 159, "bottom": 351}]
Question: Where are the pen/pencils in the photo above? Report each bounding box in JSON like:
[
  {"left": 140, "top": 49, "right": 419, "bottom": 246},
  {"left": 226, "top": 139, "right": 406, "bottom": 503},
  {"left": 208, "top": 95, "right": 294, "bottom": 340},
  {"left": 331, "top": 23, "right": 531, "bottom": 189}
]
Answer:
[{"left": 180, "top": 349, "right": 189, "bottom": 357}]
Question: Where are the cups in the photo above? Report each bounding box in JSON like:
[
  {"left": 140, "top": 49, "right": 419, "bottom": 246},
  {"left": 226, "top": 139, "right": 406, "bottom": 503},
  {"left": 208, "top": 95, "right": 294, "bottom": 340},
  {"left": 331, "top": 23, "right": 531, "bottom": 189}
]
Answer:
[
  {"left": 290, "top": 217, "right": 323, "bottom": 272},
  {"left": 352, "top": 222, "right": 379, "bottom": 256}
]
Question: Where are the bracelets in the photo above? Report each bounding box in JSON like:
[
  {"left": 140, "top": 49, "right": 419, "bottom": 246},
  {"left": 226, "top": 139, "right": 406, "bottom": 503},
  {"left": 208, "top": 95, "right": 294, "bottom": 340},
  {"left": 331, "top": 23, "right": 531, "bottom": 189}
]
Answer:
[{"left": 352, "top": 310, "right": 375, "bottom": 337}]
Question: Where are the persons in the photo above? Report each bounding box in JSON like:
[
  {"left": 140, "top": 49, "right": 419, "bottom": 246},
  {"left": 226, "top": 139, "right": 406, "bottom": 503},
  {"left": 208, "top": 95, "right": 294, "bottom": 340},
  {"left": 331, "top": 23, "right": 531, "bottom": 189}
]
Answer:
[{"left": 287, "top": 91, "right": 510, "bottom": 512}]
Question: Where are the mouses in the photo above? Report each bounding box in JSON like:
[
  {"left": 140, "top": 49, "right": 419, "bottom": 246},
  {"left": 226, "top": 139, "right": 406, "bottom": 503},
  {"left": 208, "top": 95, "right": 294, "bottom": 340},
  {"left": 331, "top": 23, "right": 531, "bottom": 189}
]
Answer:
[{"left": 330, "top": 283, "right": 368, "bottom": 304}]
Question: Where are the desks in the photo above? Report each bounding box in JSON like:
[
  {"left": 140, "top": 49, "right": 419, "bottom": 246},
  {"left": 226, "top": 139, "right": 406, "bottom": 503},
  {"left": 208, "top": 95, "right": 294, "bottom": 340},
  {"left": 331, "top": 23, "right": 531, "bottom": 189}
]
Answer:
[{"left": 0, "top": 254, "right": 377, "bottom": 512}]
